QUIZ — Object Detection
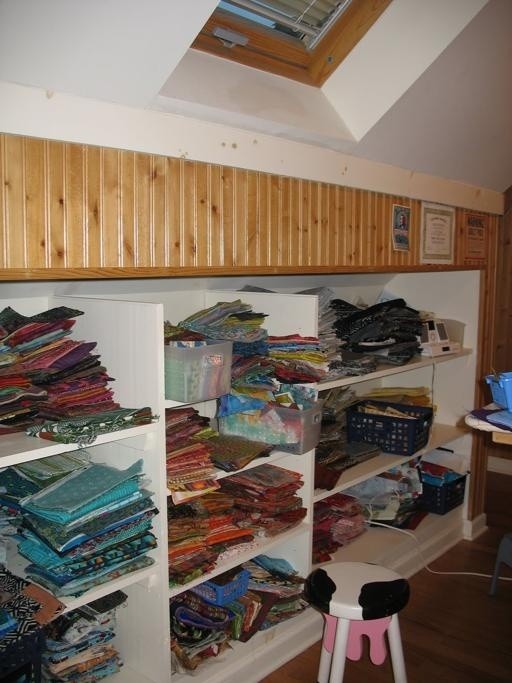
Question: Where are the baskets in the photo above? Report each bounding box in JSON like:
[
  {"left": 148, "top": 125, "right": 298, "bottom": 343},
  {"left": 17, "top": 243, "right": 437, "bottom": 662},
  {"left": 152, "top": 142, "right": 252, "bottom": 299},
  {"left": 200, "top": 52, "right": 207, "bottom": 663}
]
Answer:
[
  {"left": 485, "top": 370, "right": 512, "bottom": 411},
  {"left": 414, "top": 474, "right": 468, "bottom": 515},
  {"left": 188, "top": 569, "right": 251, "bottom": 606},
  {"left": 0, "top": 631, "right": 45, "bottom": 679},
  {"left": 342, "top": 400, "right": 433, "bottom": 456}
]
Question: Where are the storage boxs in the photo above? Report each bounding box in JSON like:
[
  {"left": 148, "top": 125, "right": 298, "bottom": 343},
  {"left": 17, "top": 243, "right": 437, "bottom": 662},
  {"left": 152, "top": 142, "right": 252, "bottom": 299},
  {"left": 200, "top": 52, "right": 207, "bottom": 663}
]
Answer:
[{"left": 164, "top": 336, "right": 321, "bottom": 455}]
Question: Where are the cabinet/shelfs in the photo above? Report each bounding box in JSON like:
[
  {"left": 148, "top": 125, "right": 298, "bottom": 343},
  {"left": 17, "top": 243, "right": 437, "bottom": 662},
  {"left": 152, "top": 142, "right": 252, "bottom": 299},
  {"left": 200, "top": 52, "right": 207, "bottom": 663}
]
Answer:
[{"left": 0, "top": 271, "right": 483, "bottom": 682}]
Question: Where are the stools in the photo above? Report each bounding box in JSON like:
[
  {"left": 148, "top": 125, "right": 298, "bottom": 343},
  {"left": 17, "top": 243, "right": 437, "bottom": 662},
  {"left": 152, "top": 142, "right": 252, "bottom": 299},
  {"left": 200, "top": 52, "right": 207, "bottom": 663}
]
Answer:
[{"left": 306, "top": 560, "right": 410, "bottom": 682}]
